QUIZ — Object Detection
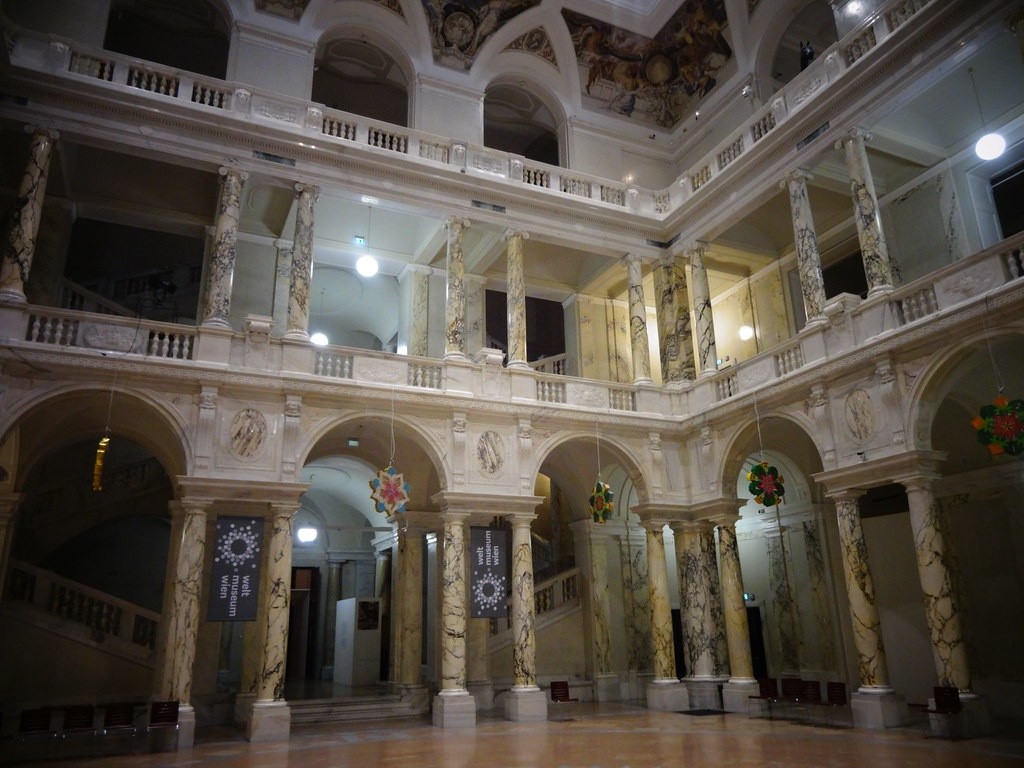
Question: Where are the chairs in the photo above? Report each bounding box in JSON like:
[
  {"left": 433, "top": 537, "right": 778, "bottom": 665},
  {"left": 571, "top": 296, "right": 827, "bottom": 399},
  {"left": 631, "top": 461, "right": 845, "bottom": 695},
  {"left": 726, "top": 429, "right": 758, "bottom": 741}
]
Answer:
[
  {"left": 920, "top": 686, "right": 962, "bottom": 742},
  {"left": 15, "top": 700, "right": 180, "bottom": 762},
  {"left": 748, "top": 678, "right": 848, "bottom": 730},
  {"left": 550, "top": 681, "right": 582, "bottom": 724}
]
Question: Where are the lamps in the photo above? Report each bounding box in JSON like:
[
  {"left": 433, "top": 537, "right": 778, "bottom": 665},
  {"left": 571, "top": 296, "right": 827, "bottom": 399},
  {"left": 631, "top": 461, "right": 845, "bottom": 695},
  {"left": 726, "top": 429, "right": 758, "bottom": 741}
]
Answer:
[
  {"left": 309, "top": 288, "right": 328, "bottom": 346},
  {"left": 356, "top": 205, "right": 378, "bottom": 278},
  {"left": 968, "top": 67, "right": 1007, "bottom": 161}
]
